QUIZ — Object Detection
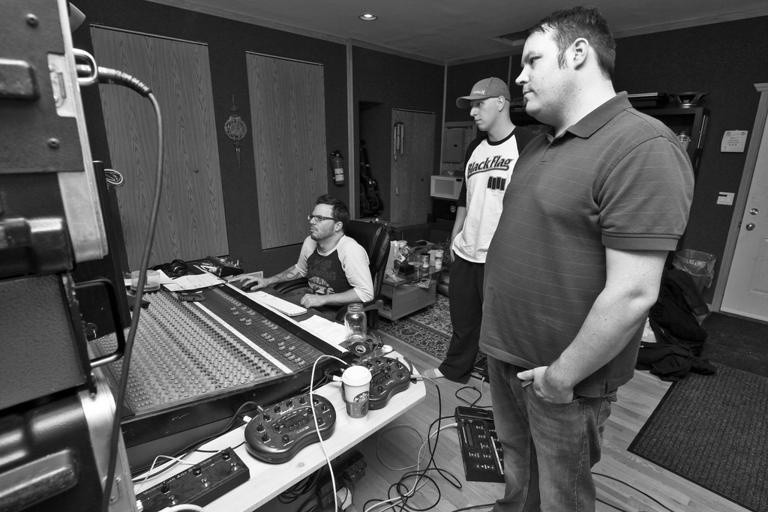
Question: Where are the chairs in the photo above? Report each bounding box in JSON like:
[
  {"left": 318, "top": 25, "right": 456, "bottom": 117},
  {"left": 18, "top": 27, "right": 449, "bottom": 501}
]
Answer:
[{"left": 275, "top": 216, "right": 392, "bottom": 333}]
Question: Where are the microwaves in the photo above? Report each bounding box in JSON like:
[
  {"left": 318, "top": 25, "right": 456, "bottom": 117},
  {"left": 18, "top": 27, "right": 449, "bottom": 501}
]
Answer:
[{"left": 429, "top": 173, "right": 464, "bottom": 201}]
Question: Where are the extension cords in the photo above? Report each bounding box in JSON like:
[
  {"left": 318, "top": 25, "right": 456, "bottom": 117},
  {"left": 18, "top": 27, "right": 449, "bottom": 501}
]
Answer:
[{"left": 317, "top": 449, "right": 366, "bottom": 510}]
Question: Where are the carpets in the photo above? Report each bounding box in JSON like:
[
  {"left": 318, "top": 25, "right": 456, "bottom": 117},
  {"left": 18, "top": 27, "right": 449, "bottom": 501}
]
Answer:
[
  {"left": 624, "top": 362, "right": 767, "bottom": 512},
  {"left": 374, "top": 234, "right": 488, "bottom": 384}
]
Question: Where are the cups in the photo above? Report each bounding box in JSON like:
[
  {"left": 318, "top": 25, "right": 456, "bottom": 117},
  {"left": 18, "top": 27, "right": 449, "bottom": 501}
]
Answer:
[
  {"left": 342, "top": 365, "right": 372, "bottom": 418},
  {"left": 421, "top": 253, "right": 430, "bottom": 269}
]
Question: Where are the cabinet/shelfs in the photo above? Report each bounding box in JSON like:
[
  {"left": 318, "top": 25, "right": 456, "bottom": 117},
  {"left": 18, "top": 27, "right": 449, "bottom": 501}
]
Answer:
[
  {"left": 360, "top": 108, "right": 438, "bottom": 231},
  {"left": 637, "top": 107, "right": 710, "bottom": 175}
]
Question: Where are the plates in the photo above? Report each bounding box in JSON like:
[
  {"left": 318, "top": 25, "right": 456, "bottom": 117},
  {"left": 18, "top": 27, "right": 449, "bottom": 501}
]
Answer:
[{"left": 669, "top": 91, "right": 711, "bottom": 108}]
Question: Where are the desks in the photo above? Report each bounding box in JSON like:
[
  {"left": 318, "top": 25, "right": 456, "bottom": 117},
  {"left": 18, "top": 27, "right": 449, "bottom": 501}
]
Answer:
[{"left": 376, "top": 230, "right": 458, "bottom": 323}]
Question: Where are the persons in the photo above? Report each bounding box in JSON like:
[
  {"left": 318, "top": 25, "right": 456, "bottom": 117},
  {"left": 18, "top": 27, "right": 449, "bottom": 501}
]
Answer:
[
  {"left": 238, "top": 193, "right": 376, "bottom": 322},
  {"left": 475, "top": 5, "right": 695, "bottom": 512},
  {"left": 421, "top": 78, "right": 543, "bottom": 384}
]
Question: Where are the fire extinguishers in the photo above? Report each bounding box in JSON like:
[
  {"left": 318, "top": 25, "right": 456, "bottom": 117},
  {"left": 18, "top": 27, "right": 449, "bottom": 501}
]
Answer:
[{"left": 329, "top": 150, "right": 345, "bottom": 186}]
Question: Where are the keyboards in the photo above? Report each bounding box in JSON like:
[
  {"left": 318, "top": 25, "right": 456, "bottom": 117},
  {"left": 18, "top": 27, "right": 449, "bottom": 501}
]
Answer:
[{"left": 251, "top": 291, "right": 308, "bottom": 317}]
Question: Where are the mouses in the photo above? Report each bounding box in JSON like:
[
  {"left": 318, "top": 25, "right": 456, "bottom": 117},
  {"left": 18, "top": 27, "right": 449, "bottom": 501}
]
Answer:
[{"left": 241, "top": 281, "right": 258, "bottom": 292}]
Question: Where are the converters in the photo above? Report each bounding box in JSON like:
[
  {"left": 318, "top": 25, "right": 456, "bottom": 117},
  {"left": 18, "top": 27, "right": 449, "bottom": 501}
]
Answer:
[{"left": 334, "top": 487, "right": 353, "bottom": 510}]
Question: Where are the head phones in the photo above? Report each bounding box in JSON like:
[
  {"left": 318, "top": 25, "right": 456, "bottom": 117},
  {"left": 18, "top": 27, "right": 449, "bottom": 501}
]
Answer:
[{"left": 166, "top": 259, "right": 187, "bottom": 278}]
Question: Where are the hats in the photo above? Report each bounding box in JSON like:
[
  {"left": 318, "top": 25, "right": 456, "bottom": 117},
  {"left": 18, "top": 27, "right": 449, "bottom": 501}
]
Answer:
[{"left": 455, "top": 77, "right": 512, "bottom": 111}]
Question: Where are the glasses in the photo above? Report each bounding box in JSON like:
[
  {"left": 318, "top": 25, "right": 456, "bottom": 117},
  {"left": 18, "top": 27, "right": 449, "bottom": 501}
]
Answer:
[{"left": 306, "top": 214, "right": 334, "bottom": 223}]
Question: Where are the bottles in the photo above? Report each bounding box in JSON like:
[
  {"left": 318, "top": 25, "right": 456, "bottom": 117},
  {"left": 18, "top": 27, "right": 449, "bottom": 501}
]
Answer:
[
  {"left": 344, "top": 303, "right": 367, "bottom": 340},
  {"left": 435, "top": 251, "right": 442, "bottom": 270}
]
